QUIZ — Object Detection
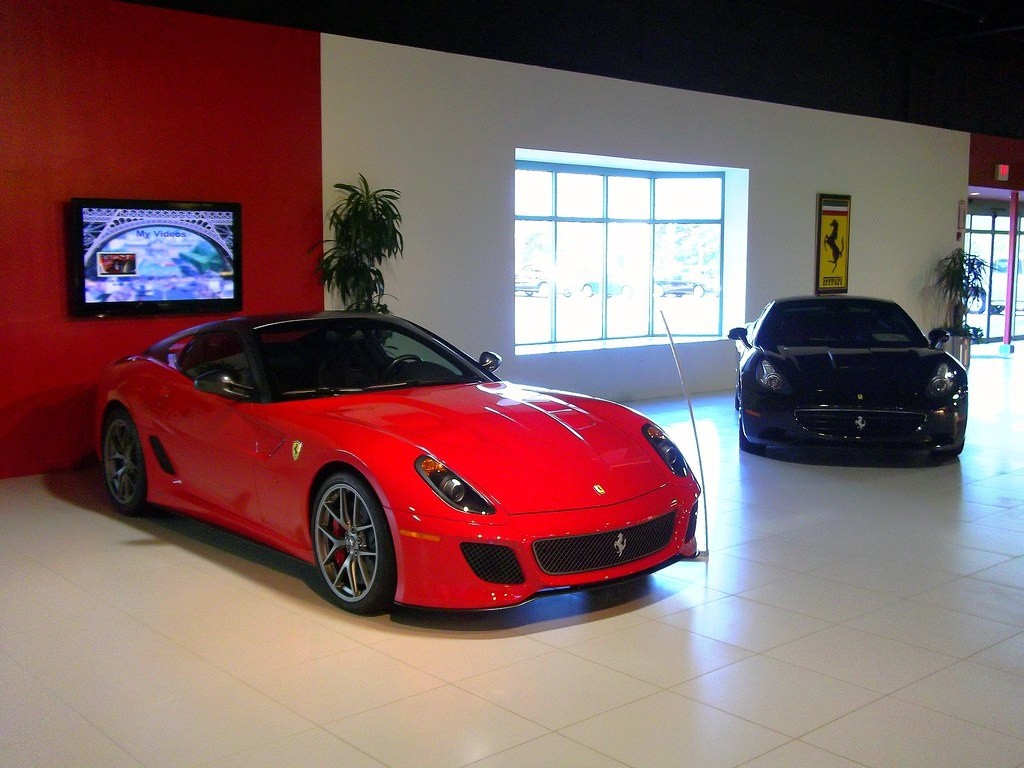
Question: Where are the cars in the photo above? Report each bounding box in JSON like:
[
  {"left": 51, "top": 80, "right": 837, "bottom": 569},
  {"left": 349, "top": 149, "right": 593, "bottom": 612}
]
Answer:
[
  {"left": 558, "top": 278, "right": 622, "bottom": 299},
  {"left": 515, "top": 264, "right": 551, "bottom": 299},
  {"left": 963, "top": 255, "right": 1023, "bottom": 316},
  {"left": 653, "top": 279, "right": 710, "bottom": 298},
  {"left": 94, "top": 304, "right": 701, "bottom": 618},
  {"left": 730, "top": 294, "right": 968, "bottom": 459}
]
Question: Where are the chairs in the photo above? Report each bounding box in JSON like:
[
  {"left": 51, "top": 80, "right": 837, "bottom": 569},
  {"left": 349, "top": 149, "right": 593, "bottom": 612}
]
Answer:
[{"left": 197, "top": 328, "right": 412, "bottom": 397}]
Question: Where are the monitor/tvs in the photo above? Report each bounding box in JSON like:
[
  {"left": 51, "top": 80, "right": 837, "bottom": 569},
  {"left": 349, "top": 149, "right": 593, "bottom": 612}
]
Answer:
[{"left": 68, "top": 197, "right": 242, "bottom": 316}]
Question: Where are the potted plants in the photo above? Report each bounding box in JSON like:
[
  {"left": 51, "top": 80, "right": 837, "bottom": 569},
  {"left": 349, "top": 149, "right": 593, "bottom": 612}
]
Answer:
[{"left": 926, "top": 247, "right": 991, "bottom": 373}]
